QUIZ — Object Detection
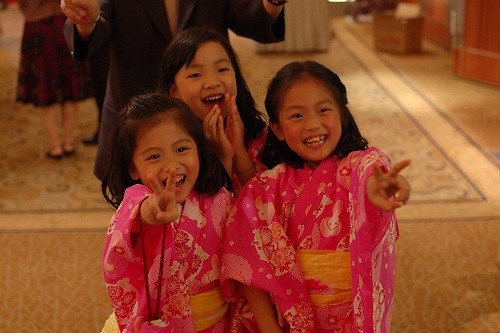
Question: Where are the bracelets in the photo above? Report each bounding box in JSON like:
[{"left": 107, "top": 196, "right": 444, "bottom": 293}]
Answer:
[
  {"left": 235, "top": 160, "right": 256, "bottom": 173},
  {"left": 79, "top": 13, "right": 100, "bottom": 26},
  {"left": 267, "top": 0, "right": 289, "bottom": 6}
]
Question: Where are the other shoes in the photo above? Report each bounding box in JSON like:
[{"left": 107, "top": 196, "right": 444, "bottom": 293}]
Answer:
[
  {"left": 62, "top": 141, "right": 76, "bottom": 155},
  {"left": 46, "top": 146, "right": 66, "bottom": 160},
  {"left": 80, "top": 128, "right": 99, "bottom": 146}
]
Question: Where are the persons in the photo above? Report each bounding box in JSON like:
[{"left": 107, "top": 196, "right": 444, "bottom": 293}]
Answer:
[{"left": 14, "top": 0, "right": 411, "bottom": 333}]
